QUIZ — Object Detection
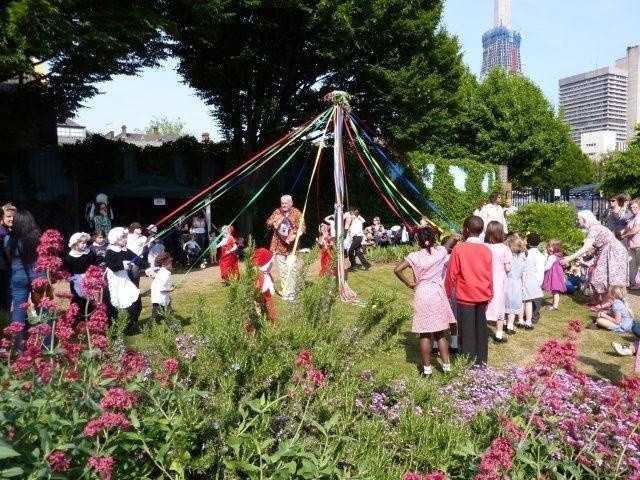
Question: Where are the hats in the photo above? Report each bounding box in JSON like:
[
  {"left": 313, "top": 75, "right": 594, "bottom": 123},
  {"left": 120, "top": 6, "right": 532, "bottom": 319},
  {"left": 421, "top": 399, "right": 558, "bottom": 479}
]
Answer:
[
  {"left": 68, "top": 231, "right": 91, "bottom": 248},
  {"left": 145, "top": 224, "right": 158, "bottom": 233},
  {"left": 107, "top": 227, "right": 129, "bottom": 245},
  {"left": 252, "top": 248, "right": 276, "bottom": 272}
]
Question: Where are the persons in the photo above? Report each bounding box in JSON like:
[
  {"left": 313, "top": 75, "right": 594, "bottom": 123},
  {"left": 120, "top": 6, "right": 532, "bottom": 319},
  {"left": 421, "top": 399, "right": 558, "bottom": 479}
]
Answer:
[
  {"left": 63, "top": 223, "right": 177, "bottom": 338},
  {"left": 590, "top": 288, "right": 640, "bottom": 356},
  {"left": 179, "top": 223, "right": 244, "bottom": 284},
  {"left": 319, "top": 208, "right": 371, "bottom": 275},
  {"left": 366, "top": 216, "right": 410, "bottom": 246},
  {"left": 1, "top": 204, "right": 51, "bottom": 368},
  {"left": 446, "top": 216, "right": 492, "bottom": 368},
  {"left": 242, "top": 248, "right": 277, "bottom": 332},
  {"left": 395, "top": 227, "right": 455, "bottom": 378},
  {"left": 441, "top": 232, "right": 566, "bottom": 352},
  {"left": 266, "top": 195, "right": 305, "bottom": 299},
  {"left": 561, "top": 193, "right": 640, "bottom": 312},
  {"left": 88, "top": 194, "right": 113, "bottom": 233},
  {"left": 474, "top": 191, "right": 516, "bottom": 234}
]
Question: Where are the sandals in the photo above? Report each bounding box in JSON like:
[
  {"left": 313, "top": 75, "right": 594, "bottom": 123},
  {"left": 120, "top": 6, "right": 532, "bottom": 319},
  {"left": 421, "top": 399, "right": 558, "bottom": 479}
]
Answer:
[{"left": 547, "top": 305, "right": 557, "bottom": 311}]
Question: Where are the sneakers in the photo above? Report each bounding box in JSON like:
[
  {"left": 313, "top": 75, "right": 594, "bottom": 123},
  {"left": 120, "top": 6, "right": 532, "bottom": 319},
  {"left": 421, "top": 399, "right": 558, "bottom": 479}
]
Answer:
[
  {"left": 496, "top": 337, "right": 509, "bottom": 344},
  {"left": 516, "top": 321, "right": 525, "bottom": 329},
  {"left": 504, "top": 324, "right": 516, "bottom": 335},
  {"left": 611, "top": 341, "right": 635, "bottom": 356},
  {"left": 423, "top": 373, "right": 432, "bottom": 379},
  {"left": 524, "top": 324, "right": 534, "bottom": 330},
  {"left": 441, "top": 366, "right": 451, "bottom": 375},
  {"left": 432, "top": 346, "right": 442, "bottom": 356},
  {"left": 282, "top": 291, "right": 296, "bottom": 300},
  {"left": 348, "top": 263, "right": 372, "bottom": 272},
  {"left": 449, "top": 346, "right": 457, "bottom": 355}
]
